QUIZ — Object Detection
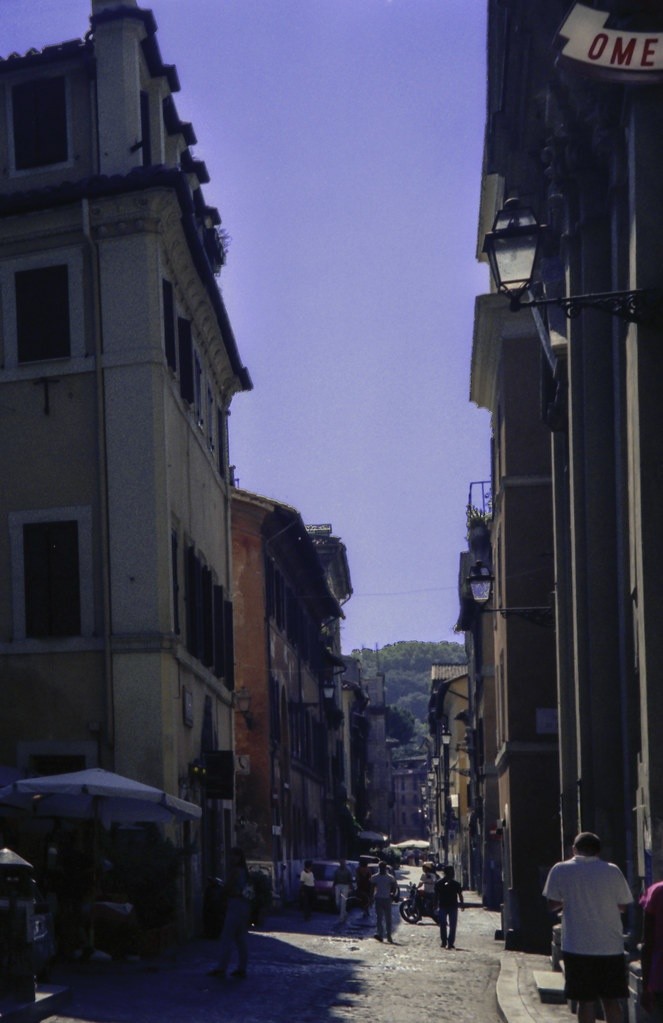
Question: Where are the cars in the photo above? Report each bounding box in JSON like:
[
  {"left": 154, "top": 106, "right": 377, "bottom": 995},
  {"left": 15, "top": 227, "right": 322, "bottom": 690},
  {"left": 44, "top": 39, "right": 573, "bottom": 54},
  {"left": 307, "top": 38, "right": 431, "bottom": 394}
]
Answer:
[
  {"left": 346, "top": 854, "right": 395, "bottom": 893},
  {"left": 298, "top": 860, "right": 357, "bottom": 909}
]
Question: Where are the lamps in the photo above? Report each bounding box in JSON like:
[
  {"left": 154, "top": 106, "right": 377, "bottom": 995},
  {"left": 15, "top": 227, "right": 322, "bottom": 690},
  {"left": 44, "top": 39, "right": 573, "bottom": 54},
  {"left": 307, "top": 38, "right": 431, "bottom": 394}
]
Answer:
[
  {"left": 421, "top": 779, "right": 432, "bottom": 818},
  {"left": 427, "top": 767, "right": 437, "bottom": 779},
  {"left": 480, "top": 197, "right": 663, "bottom": 335},
  {"left": 439, "top": 721, "right": 452, "bottom": 744},
  {"left": 430, "top": 752, "right": 440, "bottom": 766},
  {"left": 466, "top": 560, "right": 556, "bottom": 631},
  {"left": 312, "top": 670, "right": 337, "bottom": 698}
]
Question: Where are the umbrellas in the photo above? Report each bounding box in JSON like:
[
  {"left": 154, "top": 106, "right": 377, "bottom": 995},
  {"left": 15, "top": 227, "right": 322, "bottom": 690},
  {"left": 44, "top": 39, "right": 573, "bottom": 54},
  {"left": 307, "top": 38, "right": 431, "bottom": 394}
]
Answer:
[{"left": 1, "top": 768, "right": 201, "bottom": 822}]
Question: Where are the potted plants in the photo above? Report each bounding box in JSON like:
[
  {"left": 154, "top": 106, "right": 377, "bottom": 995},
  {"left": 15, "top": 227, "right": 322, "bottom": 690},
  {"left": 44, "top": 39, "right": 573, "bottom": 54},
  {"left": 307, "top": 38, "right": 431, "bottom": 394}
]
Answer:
[{"left": 465, "top": 505, "right": 492, "bottom": 552}]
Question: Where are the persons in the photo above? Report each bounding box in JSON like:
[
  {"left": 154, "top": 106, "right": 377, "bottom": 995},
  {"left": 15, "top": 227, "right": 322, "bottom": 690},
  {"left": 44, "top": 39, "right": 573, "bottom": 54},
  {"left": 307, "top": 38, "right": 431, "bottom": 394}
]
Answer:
[
  {"left": 542, "top": 832, "right": 634, "bottom": 1023},
  {"left": 334, "top": 857, "right": 353, "bottom": 922},
  {"left": 414, "top": 861, "right": 440, "bottom": 915},
  {"left": 366, "top": 840, "right": 425, "bottom": 866},
  {"left": 434, "top": 866, "right": 464, "bottom": 948},
  {"left": 639, "top": 882, "right": 662, "bottom": 1013},
  {"left": 356, "top": 859, "right": 370, "bottom": 916},
  {"left": 207, "top": 847, "right": 252, "bottom": 978},
  {"left": 54, "top": 826, "right": 93, "bottom": 947},
  {"left": 299, "top": 860, "right": 316, "bottom": 920},
  {"left": 369, "top": 861, "right": 397, "bottom": 942}
]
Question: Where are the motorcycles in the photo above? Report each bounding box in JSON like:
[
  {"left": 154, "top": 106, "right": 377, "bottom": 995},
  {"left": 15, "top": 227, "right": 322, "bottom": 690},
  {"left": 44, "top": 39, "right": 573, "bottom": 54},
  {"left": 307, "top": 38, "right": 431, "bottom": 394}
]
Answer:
[{"left": 399, "top": 881, "right": 447, "bottom": 926}]
